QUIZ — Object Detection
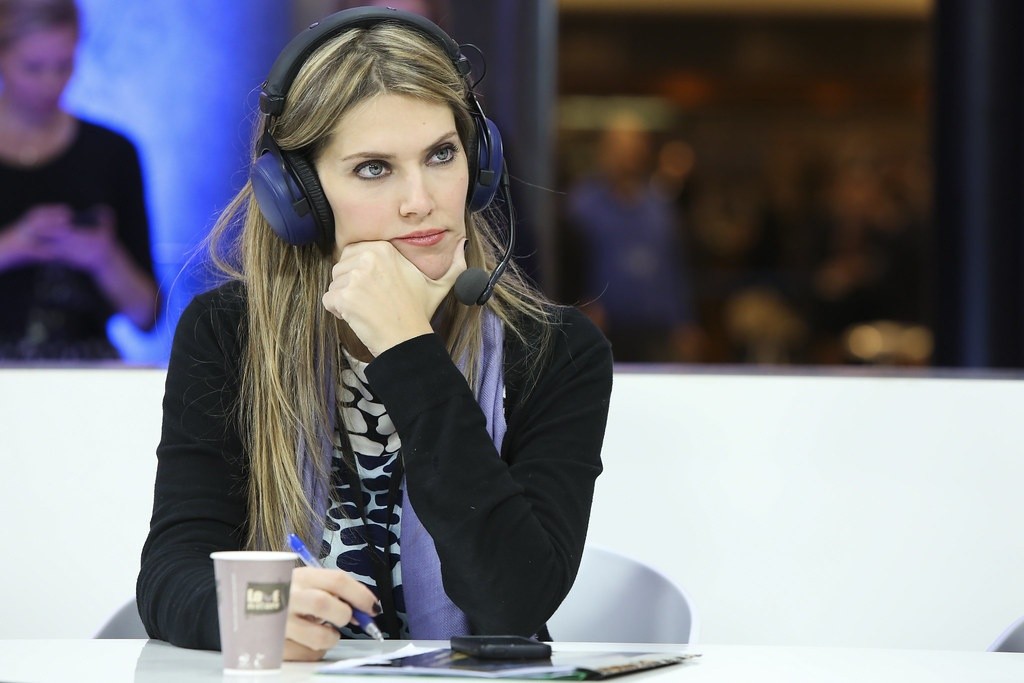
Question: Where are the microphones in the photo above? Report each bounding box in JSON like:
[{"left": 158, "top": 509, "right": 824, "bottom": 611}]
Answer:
[{"left": 453, "top": 159, "right": 515, "bottom": 306}]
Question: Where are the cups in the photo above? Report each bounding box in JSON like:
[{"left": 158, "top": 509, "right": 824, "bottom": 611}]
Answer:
[{"left": 210, "top": 550, "right": 299, "bottom": 675}]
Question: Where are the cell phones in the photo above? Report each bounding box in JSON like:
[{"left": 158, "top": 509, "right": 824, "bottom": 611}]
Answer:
[{"left": 450, "top": 635, "right": 552, "bottom": 660}]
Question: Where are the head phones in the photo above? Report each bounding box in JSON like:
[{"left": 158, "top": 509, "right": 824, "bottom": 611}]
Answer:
[{"left": 250, "top": 5, "right": 503, "bottom": 257}]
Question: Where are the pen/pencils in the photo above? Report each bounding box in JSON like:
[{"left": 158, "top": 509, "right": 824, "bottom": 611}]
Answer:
[{"left": 289, "top": 535, "right": 384, "bottom": 644}]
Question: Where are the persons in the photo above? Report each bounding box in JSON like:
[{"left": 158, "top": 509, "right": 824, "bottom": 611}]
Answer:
[
  {"left": 135, "top": 2, "right": 614, "bottom": 663},
  {"left": 0, "top": 0, "right": 163, "bottom": 364},
  {"left": 563, "top": 101, "right": 939, "bottom": 366}
]
